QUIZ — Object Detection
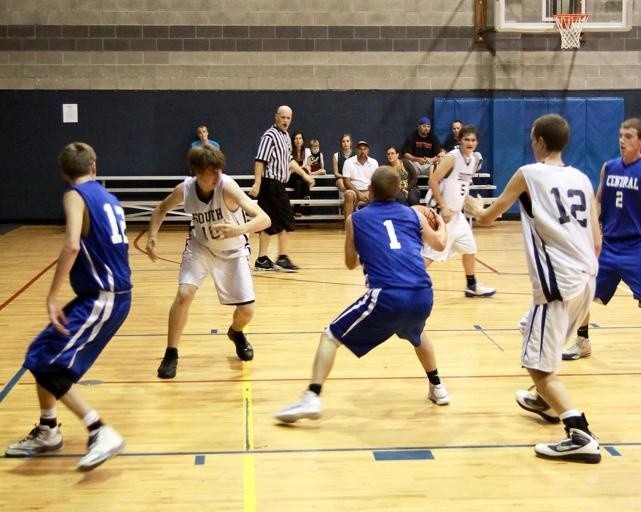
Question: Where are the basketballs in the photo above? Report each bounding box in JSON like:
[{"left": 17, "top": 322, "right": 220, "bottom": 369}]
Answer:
[{"left": 413, "top": 205, "right": 437, "bottom": 231}]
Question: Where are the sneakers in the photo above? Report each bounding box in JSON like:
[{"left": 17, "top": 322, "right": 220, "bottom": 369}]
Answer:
[
  {"left": 4, "top": 421, "right": 64, "bottom": 458},
  {"left": 275, "top": 391, "right": 322, "bottom": 424},
  {"left": 274, "top": 256, "right": 300, "bottom": 273},
  {"left": 254, "top": 257, "right": 278, "bottom": 271},
  {"left": 157, "top": 352, "right": 179, "bottom": 379},
  {"left": 75, "top": 425, "right": 126, "bottom": 473},
  {"left": 532, "top": 428, "right": 601, "bottom": 462},
  {"left": 465, "top": 282, "right": 496, "bottom": 299},
  {"left": 427, "top": 382, "right": 450, "bottom": 406},
  {"left": 227, "top": 327, "right": 254, "bottom": 361},
  {"left": 561, "top": 335, "right": 591, "bottom": 360},
  {"left": 515, "top": 389, "right": 560, "bottom": 424}
]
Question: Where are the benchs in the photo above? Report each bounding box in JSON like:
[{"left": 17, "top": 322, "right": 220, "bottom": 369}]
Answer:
[
  {"left": 95, "top": 175, "right": 192, "bottom": 222},
  {"left": 417, "top": 174, "right": 430, "bottom": 205},
  {"left": 230, "top": 175, "right": 259, "bottom": 205},
  {"left": 463, "top": 173, "right": 502, "bottom": 226},
  {"left": 285, "top": 173, "right": 345, "bottom": 224}
]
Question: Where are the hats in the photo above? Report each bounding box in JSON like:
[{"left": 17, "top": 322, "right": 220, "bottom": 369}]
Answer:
[
  {"left": 356, "top": 140, "right": 368, "bottom": 148},
  {"left": 417, "top": 116, "right": 431, "bottom": 125}
]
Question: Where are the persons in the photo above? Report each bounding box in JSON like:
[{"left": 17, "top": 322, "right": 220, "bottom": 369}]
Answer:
[
  {"left": 5, "top": 142, "right": 134, "bottom": 472},
  {"left": 191, "top": 125, "right": 220, "bottom": 152},
  {"left": 419, "top": 125, "right": 496, "bottom": 297},
  {"left": 463, "top": 114, "right": 603, "bottom": 461},
  {"left": 248, "top": 105, "right": 315, "bottom": 273},
  {"left": 561, "top": 118, "right": 641, "bottom": 360},
  {"left": 275, "top": 166, "right": 450, "bottom": 422},
  {"left": 285, "top": 117, "right": 483, "bottom": 230},
  {"left": 146, "top": 144, "right": 272, "bottom": 378}
]
